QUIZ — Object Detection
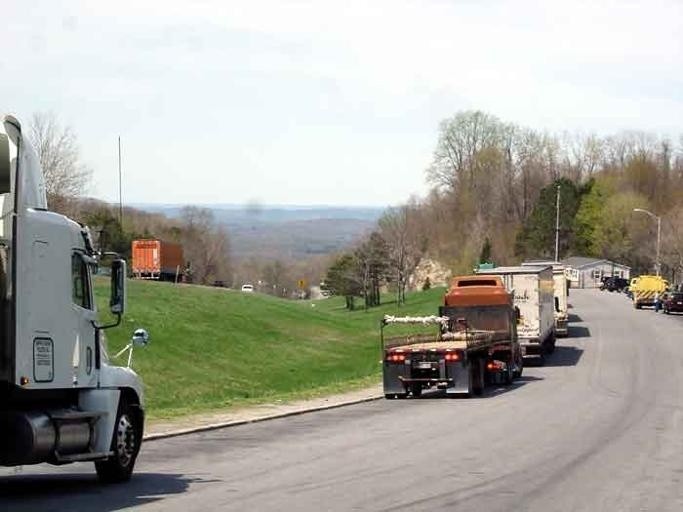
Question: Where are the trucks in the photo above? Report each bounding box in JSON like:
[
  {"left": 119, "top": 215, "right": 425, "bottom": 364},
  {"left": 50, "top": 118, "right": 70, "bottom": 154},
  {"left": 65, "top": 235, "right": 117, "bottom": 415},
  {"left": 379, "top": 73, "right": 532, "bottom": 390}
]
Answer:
[
  {"left": 0, "top": 113, "right": 149, "bottom": 482},
  {"left": 381, "top": 276, "right": 524, "bottom": 398},
  {"left": 131, "top": 240, "right": 192, "bottom": 283},
  {"left": 475, "top": 259, "right": 570, "bottom": 364}
]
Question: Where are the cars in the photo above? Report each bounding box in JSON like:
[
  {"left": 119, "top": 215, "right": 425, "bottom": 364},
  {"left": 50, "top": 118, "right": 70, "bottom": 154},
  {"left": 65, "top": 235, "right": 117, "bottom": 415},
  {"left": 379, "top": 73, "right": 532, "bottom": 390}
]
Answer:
[{"left": 600, "top": 275, "right": 683, "bottom": 313}]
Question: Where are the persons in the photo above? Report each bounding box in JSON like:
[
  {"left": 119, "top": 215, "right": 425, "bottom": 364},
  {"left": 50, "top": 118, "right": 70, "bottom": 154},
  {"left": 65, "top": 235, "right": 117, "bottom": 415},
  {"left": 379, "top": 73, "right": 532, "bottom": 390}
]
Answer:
[{"left": 654, "top": 291, "right": 660, "bottom": 311}]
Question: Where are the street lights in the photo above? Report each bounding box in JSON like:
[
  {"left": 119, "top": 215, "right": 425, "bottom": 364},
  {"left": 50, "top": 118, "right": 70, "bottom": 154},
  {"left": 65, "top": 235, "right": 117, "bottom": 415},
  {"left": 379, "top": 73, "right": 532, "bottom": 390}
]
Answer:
[{"left": 634, "top": 209, "right": 661, "bottom": 276}]
